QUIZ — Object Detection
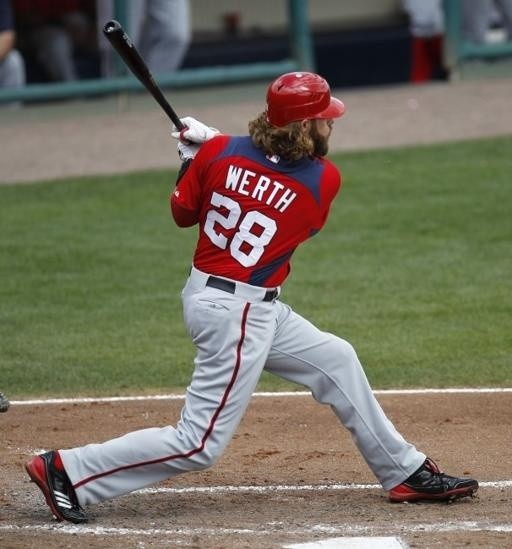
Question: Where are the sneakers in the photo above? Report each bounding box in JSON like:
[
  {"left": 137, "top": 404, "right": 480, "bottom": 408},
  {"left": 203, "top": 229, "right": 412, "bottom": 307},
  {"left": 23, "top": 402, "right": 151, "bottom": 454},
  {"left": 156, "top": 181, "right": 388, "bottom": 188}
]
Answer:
[
  {"left": 25, "top": 451, "right": 88, "bottom": 524},
  {"left": 389, "top": 464, "right": 478, "bottom": 503}
]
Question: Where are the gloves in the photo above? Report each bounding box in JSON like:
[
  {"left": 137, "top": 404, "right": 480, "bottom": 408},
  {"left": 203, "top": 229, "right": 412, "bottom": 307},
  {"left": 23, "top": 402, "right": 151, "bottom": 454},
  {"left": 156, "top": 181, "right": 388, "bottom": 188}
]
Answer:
[{"left": 171, "top": 116, "right": 223, "bottom": 162}]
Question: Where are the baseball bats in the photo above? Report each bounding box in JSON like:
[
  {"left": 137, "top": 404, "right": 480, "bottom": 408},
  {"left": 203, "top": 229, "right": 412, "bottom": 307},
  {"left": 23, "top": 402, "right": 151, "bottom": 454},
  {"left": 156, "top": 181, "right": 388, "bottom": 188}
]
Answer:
[{"left": 105, "top": 21, "right": 183, "bottom": 130}]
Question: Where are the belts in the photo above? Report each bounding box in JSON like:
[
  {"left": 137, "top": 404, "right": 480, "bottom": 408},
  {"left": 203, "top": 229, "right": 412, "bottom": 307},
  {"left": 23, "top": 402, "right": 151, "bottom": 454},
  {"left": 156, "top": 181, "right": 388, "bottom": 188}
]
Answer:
[{"left": 189, "top": 266, "right": 277, "bottom": 302}]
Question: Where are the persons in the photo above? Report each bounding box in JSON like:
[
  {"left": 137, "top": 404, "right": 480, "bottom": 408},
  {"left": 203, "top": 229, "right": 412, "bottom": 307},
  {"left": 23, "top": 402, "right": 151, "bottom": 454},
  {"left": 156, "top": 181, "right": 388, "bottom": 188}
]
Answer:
[
  {"left": 94, "top": 0, "right": 195, "bottom": 80},
  {"left": 461, "top": 0, "right": 512, "bottom": 50},
  {"left": 401, "top": 0, "right": 449, "bottom": 81},
  {"left": 23, "top": 72, "right": 478, "bottom": 522},
  {"left": 0, "top": 1, "right": 27, "bottom": 86},
  {"left": 16, "top": 2, "right": 97, "bottom": 82}
]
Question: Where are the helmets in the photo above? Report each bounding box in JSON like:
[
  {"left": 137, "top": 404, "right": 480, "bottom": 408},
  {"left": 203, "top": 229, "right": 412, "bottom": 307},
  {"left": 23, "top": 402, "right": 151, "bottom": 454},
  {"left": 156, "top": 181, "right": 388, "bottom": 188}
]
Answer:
[{"left": 266, "top": 71, "right": 345, "bottom": 130}]
